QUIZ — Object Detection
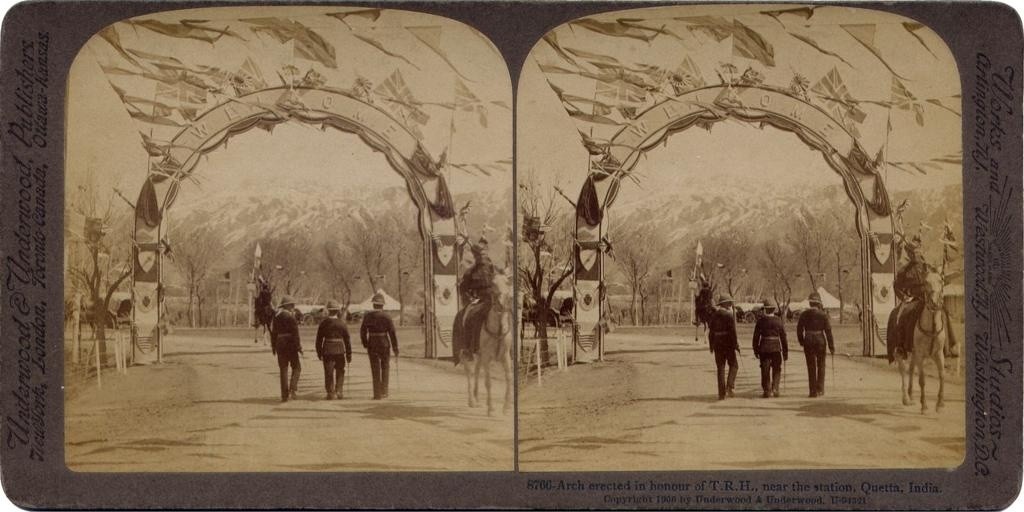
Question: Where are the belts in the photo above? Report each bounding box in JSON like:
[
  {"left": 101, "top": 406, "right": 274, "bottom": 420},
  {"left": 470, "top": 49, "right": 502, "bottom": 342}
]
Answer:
[
  {"left": 368, "top": 331, "right": 388, "bottom": 337},
  {"left": 325, "top": 338, "right": 342, "bottom": 342},
  {"left": 276, "top": 333, "right": 294, "bottom": 338},
  {"left": 761, "top": 335, "right": 781, "bottom": 339},
  {"left": 805, "top": 330, "right": 825, "bottom": 336},
  {"left": 712, "top": 331, "right": 726, "bottom": 336}
]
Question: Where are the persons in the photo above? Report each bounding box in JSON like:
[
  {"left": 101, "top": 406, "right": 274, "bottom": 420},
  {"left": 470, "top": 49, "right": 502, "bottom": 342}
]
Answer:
[
  {"left": 359, "top": 292, "right": 400, "bottom": 400},
  {"left": 270, "top": 293, "right": 304, "bottom": 402},
  {"left": 708, "top": 292, "right": 742, "bottom": 402},
  {"left": 691, "top": 281, "right": 713, "bottom": 328},
  {"left": 888, "top": 239, "right": 961, "bottom": 362},
  {"left": 752, "top": 297, "right": 789, "bottom": 399},
  {"left": 455, "top": 239, "right": 512, "bottom": 361},
  {"left": 250, "top": 282, "right": 271, "bottom": 327},
  {"left": 315, "top": 300, "right": 352, "bottom": 401},
  {"left": 795, "top": 293, "right": 835, "bottom": 397}
]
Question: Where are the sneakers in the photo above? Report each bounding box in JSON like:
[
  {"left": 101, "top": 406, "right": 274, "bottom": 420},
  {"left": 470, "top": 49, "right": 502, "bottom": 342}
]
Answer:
[
  {"left": 809, "top": 392, "right": 823, "bottom": 397},
  {"left": 282, "top": 388, "right": 295, "bottom": 402},
  {"left": 717, "top": 385, "right": 733, "bottom": 400},
  {"left": 463, "top": 350, "right": 474, "bottom": 361},
  {"left": 896, "top": 346, "right": 907, "bottom": 362},
  {"left": 763, "top": 389, "right": 780, "bottom": 397},
  {"left": 375, "top": 393, "right": 386, "bottom": 401},
  {"left": 327, "top": 391, "right": 342, "bottom": 400}
]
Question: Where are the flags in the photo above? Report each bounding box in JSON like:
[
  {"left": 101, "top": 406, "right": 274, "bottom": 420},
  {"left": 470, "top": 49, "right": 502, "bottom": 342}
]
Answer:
[
  {"left": 865, "top": 143, "right": 888, "bottom": 176},
  {"left": 594, "top": 69, "right": 648, "bottom": 106},
  {"left": 671, "top": 54, "right": 708, "bottom": 88},
  {"left": 694, "top": 239, "right": 704, "bottom": 269},
  {"left": 430, "top": 148, "right": 448, "bottom": 183},
  {"left": 453, "top": 78, "right": 489, "bottom": 128},
  {"left": 252, "top": 240, "right": 262, "bottom": 271},
  {"left": 233, "top": 54, "right": 268, "bottom": 96},
  {"left": 808, "top": 67, "right": 870, "bottom": 137},
  {"left": 597, "top": 233, "right": 616, "bottom": 262},
  {"left": 890, "top": 76, "right": 926, "bottom": 130},
  {"left": 372, "top": 66, "right": 427, "bottom": 129},
  {"left": 290, "top": 19, "right": 338, "bottom": 69},
  {"left": 730, "top": 16, "right": 776, "bottom": 68},
  {"left": 156, "top": 233, "right": 175, "bottom": 265},
  {"left": 154, "top": 82, "right": 208, "bottom": 108}
]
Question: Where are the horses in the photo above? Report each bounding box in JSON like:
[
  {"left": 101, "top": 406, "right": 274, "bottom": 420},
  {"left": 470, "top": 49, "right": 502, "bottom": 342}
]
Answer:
[
  {"left": 694, "top": 293, "right": 717, "bottom": 344},
  {"left": 458, "top": 264, "right": 514, "bottom": 417},
  {"left": 250, "top": 295, "right": 277, "bottom": 346},
  {"left": 892, "top": 262, "right": 946, "bottom": 416}
]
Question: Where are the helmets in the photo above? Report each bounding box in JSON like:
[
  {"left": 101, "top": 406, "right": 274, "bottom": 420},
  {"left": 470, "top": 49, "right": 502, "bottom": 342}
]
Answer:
[
  {"left": 716, "top": 293, "right": 735, "bottom": 304},
  {"left": 807, "top": 293, "right": 822, "bottom": 304},
  {"left": 370, "top": 293, "right": 386, "bottom": 305},
  {"left": 763, "top": 298, "right": 776, "bottom": 308},
  {"left": 327, "top": 300, "right": 342, "bottom": 312},
  {"left": 277, "top": 295, "right": 296, "bottom": 308}
]
